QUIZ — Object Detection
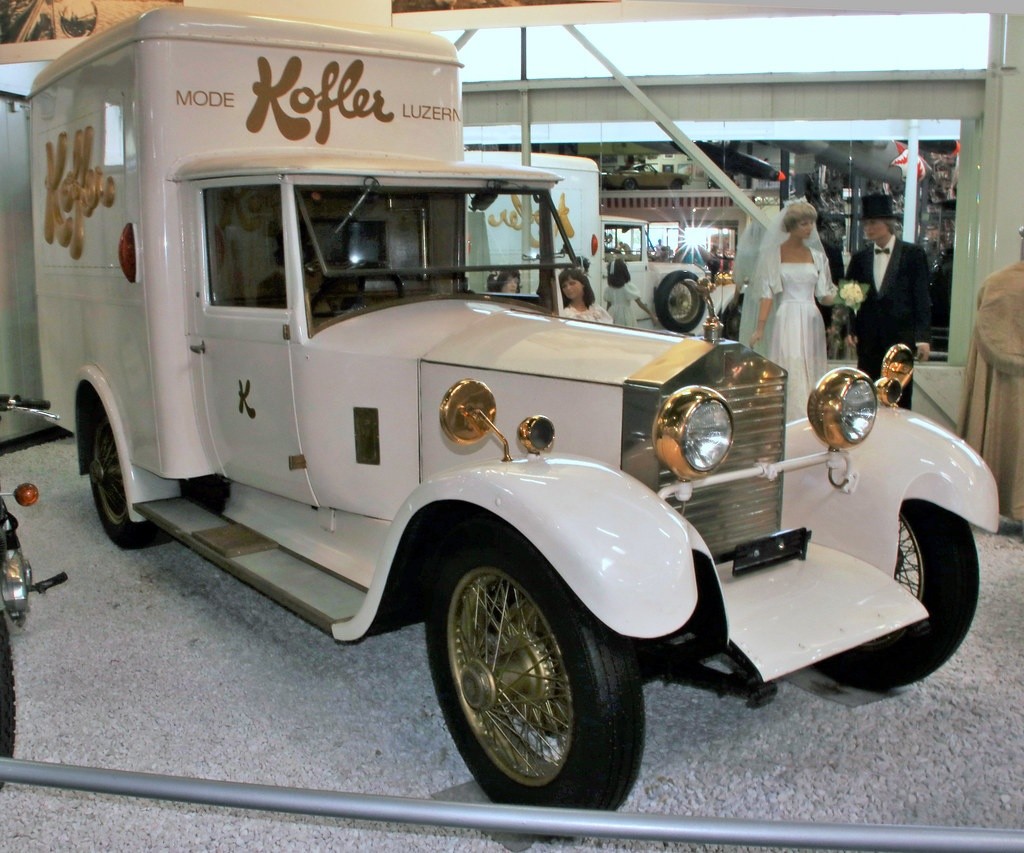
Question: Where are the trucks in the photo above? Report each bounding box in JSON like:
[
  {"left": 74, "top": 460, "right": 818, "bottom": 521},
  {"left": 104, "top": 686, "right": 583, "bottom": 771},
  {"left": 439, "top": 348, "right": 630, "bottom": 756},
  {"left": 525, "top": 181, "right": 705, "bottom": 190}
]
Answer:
[{"left": 19, "top": 10, "right": 1004, "bottom": 816}]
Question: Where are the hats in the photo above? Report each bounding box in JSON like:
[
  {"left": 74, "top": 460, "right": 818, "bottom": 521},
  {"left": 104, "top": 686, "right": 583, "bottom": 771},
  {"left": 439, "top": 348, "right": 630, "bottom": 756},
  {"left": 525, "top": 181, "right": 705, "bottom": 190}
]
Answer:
[{"left": 853, "top": 193, "right": 901, "bottom": 220}]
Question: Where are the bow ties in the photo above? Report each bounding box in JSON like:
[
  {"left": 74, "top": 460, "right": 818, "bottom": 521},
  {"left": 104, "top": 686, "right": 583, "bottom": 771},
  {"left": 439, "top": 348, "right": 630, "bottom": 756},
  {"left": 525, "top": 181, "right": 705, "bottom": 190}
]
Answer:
[{"left": 873, "top": 248, "right": 890, "bottom": 256}]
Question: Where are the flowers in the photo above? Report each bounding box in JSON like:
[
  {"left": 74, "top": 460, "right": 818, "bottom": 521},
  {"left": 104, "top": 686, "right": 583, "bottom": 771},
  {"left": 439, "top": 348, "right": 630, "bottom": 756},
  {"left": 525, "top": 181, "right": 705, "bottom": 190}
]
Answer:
[{"left": 832, "top": 278, "right": 872, "bottom": 316}]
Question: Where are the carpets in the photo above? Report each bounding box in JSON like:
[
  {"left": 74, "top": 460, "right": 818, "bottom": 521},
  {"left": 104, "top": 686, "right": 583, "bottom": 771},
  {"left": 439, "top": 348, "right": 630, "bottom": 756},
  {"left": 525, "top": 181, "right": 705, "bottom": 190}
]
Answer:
[{"left": 0, "top": 364, "right": 1024, "bottom": 852}]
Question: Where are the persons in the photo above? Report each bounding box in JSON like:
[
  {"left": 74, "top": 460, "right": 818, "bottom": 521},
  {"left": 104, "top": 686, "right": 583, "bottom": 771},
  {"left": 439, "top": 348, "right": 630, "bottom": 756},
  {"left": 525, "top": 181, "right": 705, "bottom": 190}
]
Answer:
[
  {"left": 559, "top": 269, "right": 614, "bottom": 326},
  {"left": 488, "top": 268, "right": 521, "bottom": 295},
  {"left": 604, "top": 259, "right": 659, "bottom": 329},
  {"left": 739, "top": 195, "right": 864, "bottom": 424},
  {"left": 839, "top": 194, "right": 933, "bottom": 408}
]
